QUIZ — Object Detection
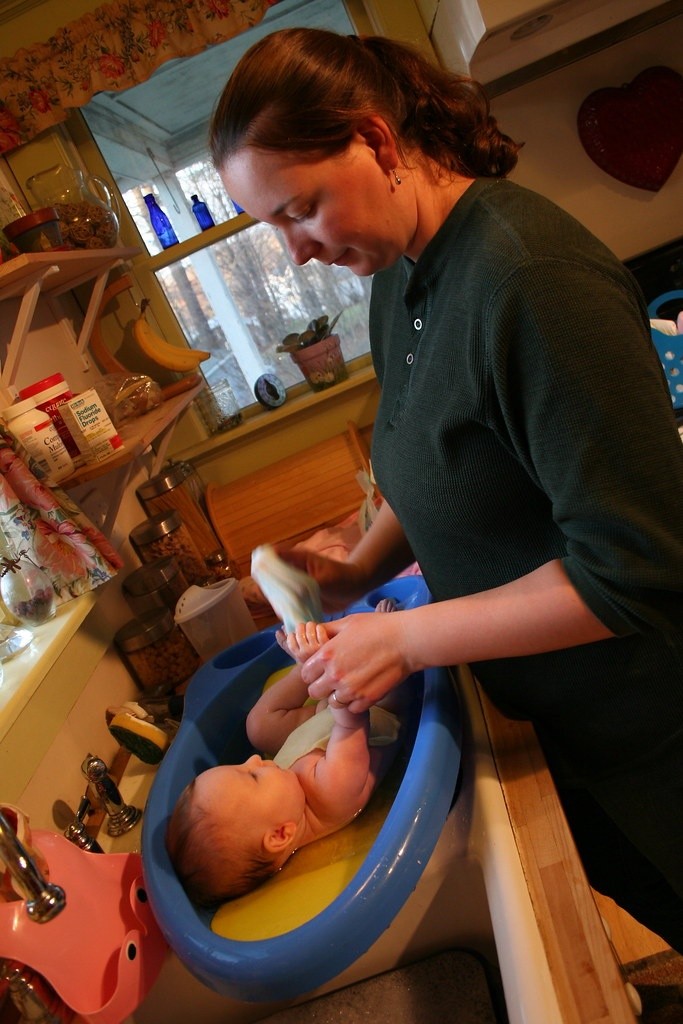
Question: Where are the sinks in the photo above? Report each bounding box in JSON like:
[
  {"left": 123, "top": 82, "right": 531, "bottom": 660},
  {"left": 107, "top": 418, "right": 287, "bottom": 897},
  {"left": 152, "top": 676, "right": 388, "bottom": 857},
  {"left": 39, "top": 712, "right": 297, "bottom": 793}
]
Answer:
[{"left": 90, "top": 834, "right": 560, "bottom": 1024}]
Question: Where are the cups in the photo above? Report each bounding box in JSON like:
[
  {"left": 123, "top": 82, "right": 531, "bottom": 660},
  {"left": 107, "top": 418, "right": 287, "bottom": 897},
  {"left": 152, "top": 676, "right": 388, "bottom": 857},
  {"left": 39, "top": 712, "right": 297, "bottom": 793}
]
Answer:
[
  {"left": 233, "top": 200, "right": 244, "bottom": 214},
  {"left": 195, "top": 378, "right": 243, "bottom": 436}
]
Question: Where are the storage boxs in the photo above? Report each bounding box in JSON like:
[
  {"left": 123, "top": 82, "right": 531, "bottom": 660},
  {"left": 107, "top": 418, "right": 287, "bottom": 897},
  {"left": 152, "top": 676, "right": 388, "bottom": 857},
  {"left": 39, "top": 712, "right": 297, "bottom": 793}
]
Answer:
[{"left": 56, "top": 386, "right": 124, "bottom": 464}]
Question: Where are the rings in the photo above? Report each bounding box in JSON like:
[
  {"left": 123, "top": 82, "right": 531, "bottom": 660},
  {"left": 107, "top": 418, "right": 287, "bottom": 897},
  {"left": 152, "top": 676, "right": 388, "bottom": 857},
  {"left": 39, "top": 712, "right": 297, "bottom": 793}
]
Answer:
[{"left": 333, "top": 692, "right": 348, "bottom": 705}]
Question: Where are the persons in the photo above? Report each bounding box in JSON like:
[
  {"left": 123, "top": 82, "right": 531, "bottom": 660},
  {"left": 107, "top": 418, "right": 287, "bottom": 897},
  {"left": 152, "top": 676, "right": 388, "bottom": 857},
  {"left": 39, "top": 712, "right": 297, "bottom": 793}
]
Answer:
[
  {"left": 208, "top": 27, "right": 683, "bottom": 959},
  {"left": 165, "top": 598, "right": 399, "bottom": 913}
]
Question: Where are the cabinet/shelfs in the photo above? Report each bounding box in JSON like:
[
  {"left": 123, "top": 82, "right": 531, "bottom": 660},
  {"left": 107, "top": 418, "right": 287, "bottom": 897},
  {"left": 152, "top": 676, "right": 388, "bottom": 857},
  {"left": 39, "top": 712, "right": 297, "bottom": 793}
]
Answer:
[
  {"left": 1, "top": 246, "right": 206, "bottom": 542},
  {"left": 417, "top": 0, "right": 683, "bottom": 100}
]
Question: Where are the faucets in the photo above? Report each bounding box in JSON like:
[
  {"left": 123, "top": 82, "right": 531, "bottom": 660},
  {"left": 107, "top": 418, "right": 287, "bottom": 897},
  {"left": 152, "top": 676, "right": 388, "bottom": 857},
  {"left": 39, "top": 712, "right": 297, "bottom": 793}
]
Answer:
[{"left": 0, "top": 815, "right": 66, "bottom": 924}]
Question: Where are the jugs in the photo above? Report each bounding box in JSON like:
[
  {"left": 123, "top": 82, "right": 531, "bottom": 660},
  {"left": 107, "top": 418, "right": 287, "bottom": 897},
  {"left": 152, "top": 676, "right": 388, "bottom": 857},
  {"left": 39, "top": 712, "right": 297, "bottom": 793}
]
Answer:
[{"left": 25, "top": 163, "right": 120, "bottom": 250}]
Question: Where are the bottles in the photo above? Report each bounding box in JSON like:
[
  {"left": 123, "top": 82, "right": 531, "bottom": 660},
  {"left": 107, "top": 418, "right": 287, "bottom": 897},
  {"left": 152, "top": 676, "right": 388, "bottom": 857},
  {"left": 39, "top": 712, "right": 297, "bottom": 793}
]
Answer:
[
  {"left": 190, "top": 195, "right": 215, "bottom": 230},
  {"left": 144, "top": 194, "right": 178, "bottom": 251}
]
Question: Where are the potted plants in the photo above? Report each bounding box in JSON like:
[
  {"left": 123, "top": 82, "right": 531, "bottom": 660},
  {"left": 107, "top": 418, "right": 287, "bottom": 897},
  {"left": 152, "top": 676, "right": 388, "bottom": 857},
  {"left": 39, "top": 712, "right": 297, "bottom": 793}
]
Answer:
[{"left": 277, "top": 311, "right": 357, "bottom": 391}]
